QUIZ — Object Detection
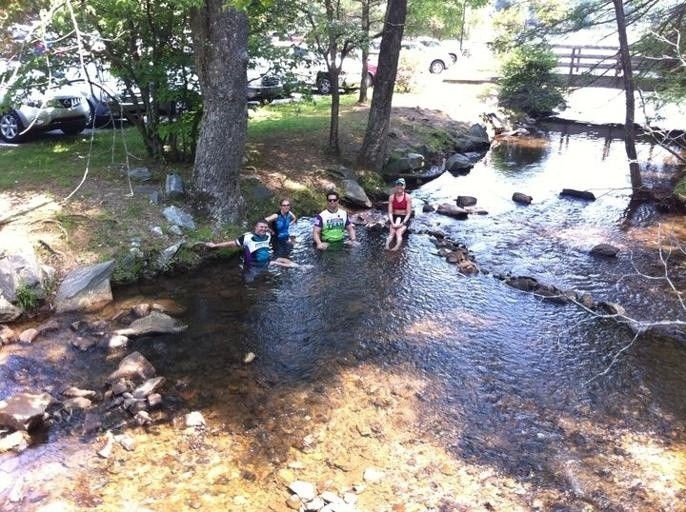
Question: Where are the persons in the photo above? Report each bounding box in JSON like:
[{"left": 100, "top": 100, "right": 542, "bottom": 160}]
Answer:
[
  {"left": 383, "top": 178, "right": 414, "bottom": 251},
  {"left": 312, "top": 192, "right": 362, "bottom": 251},
  {"left": 263, "top": 198, "right": 297, "bottom": 243},
  {"left": 268, "top": 256, "right": 314, "bottom": 273},
  {"left": 204, "top": 219, "right": 273, "bottom": 277}
]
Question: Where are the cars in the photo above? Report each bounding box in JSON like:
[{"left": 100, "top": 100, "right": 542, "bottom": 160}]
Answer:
[{"left": 0, "top": 34, "right": 461, "bottom": 144}]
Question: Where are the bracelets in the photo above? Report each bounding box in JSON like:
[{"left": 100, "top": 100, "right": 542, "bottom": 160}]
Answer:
[
  {"left": 402, "top": 221, "right": 405, "bottom": 225},
  {"left": 351, "top": 239, "right": 357, "bottom": 242}
]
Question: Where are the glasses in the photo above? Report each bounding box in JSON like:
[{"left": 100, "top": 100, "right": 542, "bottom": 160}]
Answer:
[
  {"left": 328, "top": 199, "right": 336, "bottom": 202},
  {"left": 283, "top": 204, "right": 289, "bottom": 206}
]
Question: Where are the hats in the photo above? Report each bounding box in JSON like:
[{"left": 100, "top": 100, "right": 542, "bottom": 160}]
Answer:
[{"left": 396, "top": 179, "right": 405, "bottom": 185}]
